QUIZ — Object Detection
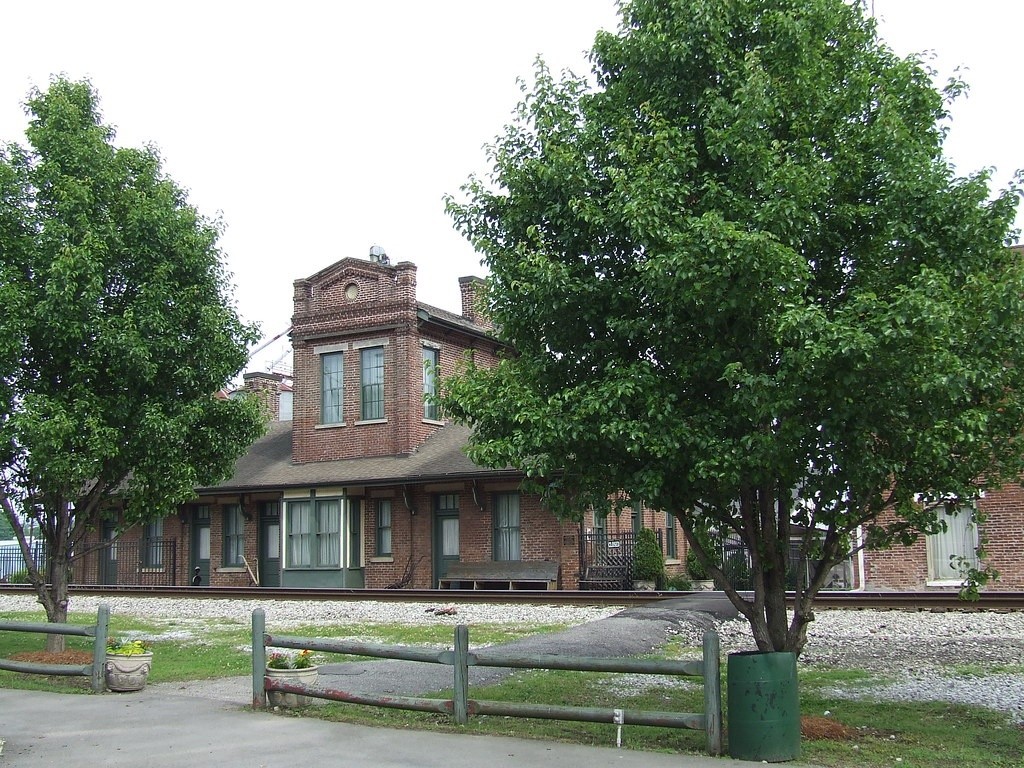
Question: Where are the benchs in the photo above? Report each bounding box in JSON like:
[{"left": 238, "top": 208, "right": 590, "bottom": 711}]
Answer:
[
  {"left": 577, "top": 565, "right": 631, "bottom": 591},
  {"left": 439, "top": 560, "right": 558, "bottom": 590}
]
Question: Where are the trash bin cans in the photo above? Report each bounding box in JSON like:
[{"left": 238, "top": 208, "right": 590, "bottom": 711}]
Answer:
[{"left": 727, "top": 651, "right": 800, "bottom": 762}]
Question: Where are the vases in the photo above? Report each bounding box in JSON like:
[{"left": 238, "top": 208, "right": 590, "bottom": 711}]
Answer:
[
  {"left": 105, "top": 651, "right": 153, "bottom": 691},
  {"left": 265, "top": 660, "right": 319, "bottom": 707}
]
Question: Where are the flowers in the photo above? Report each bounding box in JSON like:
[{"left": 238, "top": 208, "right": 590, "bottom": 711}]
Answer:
[
  {"left": 270, "top": 651, "right": 314, "bottom": 670},
  {"left": 106, "top": 637, "right": 148, "bottom": 655}
]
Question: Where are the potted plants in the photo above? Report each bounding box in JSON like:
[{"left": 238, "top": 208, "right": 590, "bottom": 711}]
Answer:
[
  {"left": 632, "top": 528, "right": 660, "bottom": 591},
  {"left": 686, "top": 514, "right": 720, "bottom": 591},
  {"left": 668, "top": 573, "right": 692, "bottom": 591}
]
